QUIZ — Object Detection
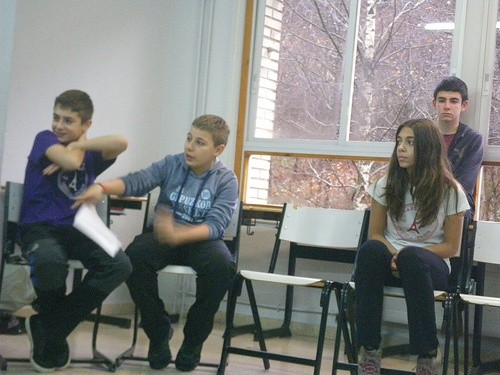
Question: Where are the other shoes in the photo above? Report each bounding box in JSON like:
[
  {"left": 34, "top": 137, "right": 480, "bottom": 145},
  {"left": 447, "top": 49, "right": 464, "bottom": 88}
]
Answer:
[
  {"left": 175, "top": 340, "right": 201, "bottom": 371},
  {"left": 56, "top": 338, "right": 70, "bottom": 370},
  {"left": 25, "top": 314, "right": 55, "bottom": 372},
  {"left": 148, "top": 327, "right": 173, "bottom": 369}
]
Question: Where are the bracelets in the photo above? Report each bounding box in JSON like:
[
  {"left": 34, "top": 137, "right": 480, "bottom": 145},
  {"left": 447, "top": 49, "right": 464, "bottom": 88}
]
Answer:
[{"left": 94, "top": 182, "right": 108, "bottom": 194}]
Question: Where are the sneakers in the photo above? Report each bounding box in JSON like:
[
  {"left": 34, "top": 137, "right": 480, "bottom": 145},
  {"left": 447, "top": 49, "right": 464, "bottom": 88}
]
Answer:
[
  {"left": 358, "top": 343, "right": 381, "bottom": 375},
  {"left": 417, "top": 347, "right": 441, "bottom": 375}
]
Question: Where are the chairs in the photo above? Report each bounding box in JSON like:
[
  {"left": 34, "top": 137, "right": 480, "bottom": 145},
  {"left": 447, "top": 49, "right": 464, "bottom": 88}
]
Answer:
[
  {"left": 332, "top": 209, "right": 472, "bottom": 375},
  {"left": 0, "top": 181, "right": 115, "bottom": 372},
  {"left": 442, "top": 220, "right": 500, "bottom": 375},
  {"left": 217, "top": 203, "right": 370, "bottom": 375},
  {"left": 114, "top": 192, "right": 243, "bottom": 367}
]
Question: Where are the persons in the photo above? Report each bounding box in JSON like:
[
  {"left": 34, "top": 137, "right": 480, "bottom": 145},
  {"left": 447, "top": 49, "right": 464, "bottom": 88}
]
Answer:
[
  {"left": 17, "top": 89, "right": 132, "bottom": 373},
  {"left": 70, "top": 115, "right": 238, "bottom": 372},
  {"left": 355, "top": 118, "right": 470, "bottom": 375},
  {"left": 433, "top": 76, "right": 485, "bottom": 220}
]
